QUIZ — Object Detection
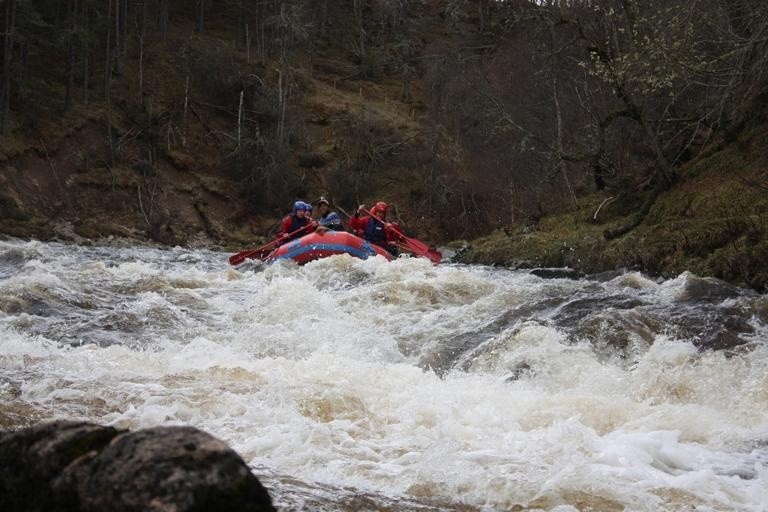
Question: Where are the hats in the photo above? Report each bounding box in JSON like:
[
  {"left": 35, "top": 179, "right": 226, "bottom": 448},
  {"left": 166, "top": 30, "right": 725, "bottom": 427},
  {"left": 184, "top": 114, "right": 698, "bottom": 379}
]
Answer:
[{"left": 318, "top": 199, "right": 330, "bottom": 206}]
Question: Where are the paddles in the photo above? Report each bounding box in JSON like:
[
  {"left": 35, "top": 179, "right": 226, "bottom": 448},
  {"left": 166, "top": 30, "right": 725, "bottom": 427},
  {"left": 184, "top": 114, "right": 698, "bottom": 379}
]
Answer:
[
  {"left": 229, "top": 221, "right": 313, "bottom": 264},
  {"left": 390, "top": 242, "right": 442, "bottom": 264},
  {"left": 363, "top": 208, "right": 428, "bottom": 255}
]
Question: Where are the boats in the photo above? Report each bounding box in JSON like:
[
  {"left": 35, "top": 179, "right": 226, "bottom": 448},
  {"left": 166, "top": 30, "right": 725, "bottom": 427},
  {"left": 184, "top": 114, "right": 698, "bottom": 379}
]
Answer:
[{"left": 268, "top": 230, "right": 393, "bottom": 269}]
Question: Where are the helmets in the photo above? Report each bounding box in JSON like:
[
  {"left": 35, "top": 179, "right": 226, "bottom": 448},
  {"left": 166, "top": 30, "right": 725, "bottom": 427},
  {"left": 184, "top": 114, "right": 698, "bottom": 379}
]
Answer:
[
  {"left": 370, "top": 202, "right": 387, "bottom": 216},
  {"left": 293, "top": 201, "right": 312, "bottom": 214}
]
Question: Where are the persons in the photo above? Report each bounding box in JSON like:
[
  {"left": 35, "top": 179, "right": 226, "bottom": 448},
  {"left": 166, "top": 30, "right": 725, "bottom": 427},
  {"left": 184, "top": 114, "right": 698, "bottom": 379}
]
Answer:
[{"left": 278, "top": 199, "right": 401, "bottom": 257}]
